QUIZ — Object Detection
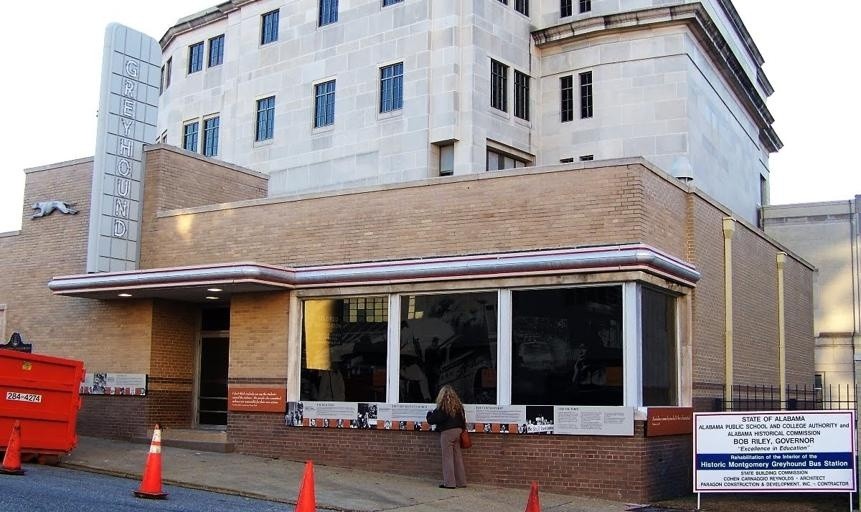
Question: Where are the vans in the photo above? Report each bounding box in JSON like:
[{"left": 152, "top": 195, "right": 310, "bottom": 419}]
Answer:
[
  {"left": 519, "top": 341, "right": 558, "bottom": 372},
  {"left": 436, "top": 335, "right": 496, "bottom": 399}
]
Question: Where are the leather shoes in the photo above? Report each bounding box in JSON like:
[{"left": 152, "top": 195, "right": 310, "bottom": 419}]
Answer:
[{"left": 439, "top": 483, "right": 466, "bottom": 489}]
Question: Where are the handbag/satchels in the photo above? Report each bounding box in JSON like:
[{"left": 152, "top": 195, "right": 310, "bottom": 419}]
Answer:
[{"left": 460, "top": 429, "right": 472, "bottom": 449}]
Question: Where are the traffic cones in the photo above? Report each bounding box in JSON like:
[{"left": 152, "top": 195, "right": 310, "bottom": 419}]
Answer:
[
  {"left": 0, "top": 419, "right": 29, "bottom": 475},
  {"left": 135, "top": 424, "right": 170, "bottom": 497},
  {"left": 525, "top": 481, "right": 541, "bottom": 512},
  {"left": 293, "top": 459, "right": 317, "bottom": 511}
]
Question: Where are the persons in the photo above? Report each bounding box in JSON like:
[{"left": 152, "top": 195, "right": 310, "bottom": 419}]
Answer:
[
  {"left": 427, "top": 384, "right": 467, "bottom": 489},
  {"left": 570, "top": 337, "right": 608, "bottom": 399}
]
